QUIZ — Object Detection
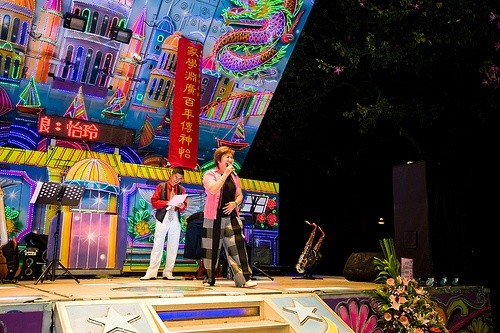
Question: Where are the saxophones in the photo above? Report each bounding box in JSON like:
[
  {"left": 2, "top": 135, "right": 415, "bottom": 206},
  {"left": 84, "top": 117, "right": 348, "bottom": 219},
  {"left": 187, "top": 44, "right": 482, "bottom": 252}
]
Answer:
[{"left": 295, "top": 219, "right": 325, "bottom": 274}]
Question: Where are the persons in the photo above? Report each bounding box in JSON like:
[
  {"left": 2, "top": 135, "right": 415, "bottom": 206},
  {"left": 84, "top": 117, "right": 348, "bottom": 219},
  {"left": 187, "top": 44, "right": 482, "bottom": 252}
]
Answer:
[
  {"left": 201, "top": 146, "right": 257, "bottom": 287},
  {"left": 140, "top": 168, "right": 187, "bottom": 280}
]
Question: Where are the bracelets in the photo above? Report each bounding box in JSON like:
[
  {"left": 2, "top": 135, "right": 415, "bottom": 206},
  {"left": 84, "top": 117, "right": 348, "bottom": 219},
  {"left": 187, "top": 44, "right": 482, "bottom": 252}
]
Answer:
[{"left": 235, "top": 201, "right": 238, "bottom": 208}]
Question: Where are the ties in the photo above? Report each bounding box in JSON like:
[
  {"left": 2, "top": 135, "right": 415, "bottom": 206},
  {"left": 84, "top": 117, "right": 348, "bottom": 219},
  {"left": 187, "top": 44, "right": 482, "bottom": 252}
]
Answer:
[{"left": 168, "top": 187, "right": 175, "bottom": 222}]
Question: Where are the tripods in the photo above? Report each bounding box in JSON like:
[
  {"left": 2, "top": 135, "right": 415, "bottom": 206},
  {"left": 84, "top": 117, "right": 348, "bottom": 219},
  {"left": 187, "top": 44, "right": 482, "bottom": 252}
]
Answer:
[
  {"left": 239, "top": 195, "right": 274, "bottom": 280},
  {"left": 33, "top": 181, "right": 85, "bottom": 285}
]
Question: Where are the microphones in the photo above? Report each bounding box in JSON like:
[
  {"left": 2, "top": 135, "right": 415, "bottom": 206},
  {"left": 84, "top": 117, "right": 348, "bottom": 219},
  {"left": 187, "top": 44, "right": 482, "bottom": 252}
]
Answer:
[{"left": 226, "top": 162, "right": 237, "bottom": 176}]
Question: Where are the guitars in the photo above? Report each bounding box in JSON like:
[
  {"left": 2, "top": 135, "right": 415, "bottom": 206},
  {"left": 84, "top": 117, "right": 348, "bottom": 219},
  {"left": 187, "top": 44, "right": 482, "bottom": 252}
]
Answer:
[{"left": 0, "top": 189, "right": 20, "bottom": 278}]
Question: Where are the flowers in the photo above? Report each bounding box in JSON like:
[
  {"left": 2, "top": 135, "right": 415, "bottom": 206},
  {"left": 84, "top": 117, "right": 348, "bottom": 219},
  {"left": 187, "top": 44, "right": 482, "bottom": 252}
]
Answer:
[{"left": 361, "top": 238, "right": 449, "bottom": 333}]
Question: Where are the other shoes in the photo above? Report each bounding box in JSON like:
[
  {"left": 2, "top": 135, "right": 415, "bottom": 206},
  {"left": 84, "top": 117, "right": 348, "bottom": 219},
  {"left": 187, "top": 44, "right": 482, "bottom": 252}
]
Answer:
[
  {"left": 163, "top": 275, "right": 175, "bottom": 280},
  {"left": 203, "top": 282, "right": 210, "bottom": 287},
  {"left": 140, "top": 275, "right": 156, "bottom": 280},
  {"left": 241, "top": 279, "right": 257, "bottom": 287}
]
